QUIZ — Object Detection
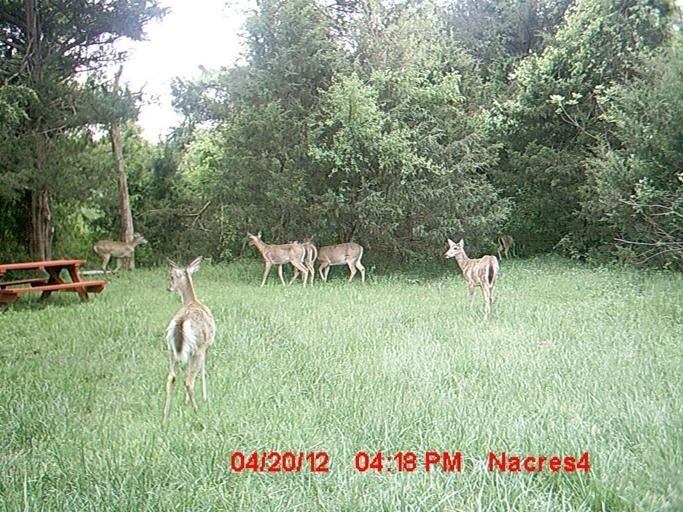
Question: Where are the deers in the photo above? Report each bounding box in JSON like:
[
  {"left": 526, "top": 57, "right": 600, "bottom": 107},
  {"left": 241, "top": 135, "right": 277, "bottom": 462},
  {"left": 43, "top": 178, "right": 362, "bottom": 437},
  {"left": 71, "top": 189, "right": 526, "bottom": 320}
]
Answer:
[
  {"left": 161, "top": 255, "right": 217, "bottom": 430},
  {"left": 314, "top": 242, "right": 367, "bottom": 284},
  {"left": 497, "top": 231, "right": 516, "bottom": 262},
  {"left": 246, "top": 229, "right": 317, "bottom": 289},
  {"left": 93, "top": 233, "right": 149, "bottom": 281},
  {"left": 444, "top": 236, "right": 501, "bottom": 320}
]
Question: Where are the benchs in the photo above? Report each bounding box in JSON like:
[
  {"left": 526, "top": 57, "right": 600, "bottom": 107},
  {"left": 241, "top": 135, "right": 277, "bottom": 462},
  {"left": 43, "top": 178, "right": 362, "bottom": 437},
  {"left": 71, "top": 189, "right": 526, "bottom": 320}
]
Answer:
[{"left": 0, "top": 277, "right": 106, "bottom": 311}]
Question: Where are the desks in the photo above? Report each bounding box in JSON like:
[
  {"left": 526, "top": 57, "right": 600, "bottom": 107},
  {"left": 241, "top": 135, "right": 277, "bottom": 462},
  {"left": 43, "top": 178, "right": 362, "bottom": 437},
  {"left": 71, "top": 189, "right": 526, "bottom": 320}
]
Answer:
[{"left": 0, "top": 259, "right": 88, "bottom": 297}]
